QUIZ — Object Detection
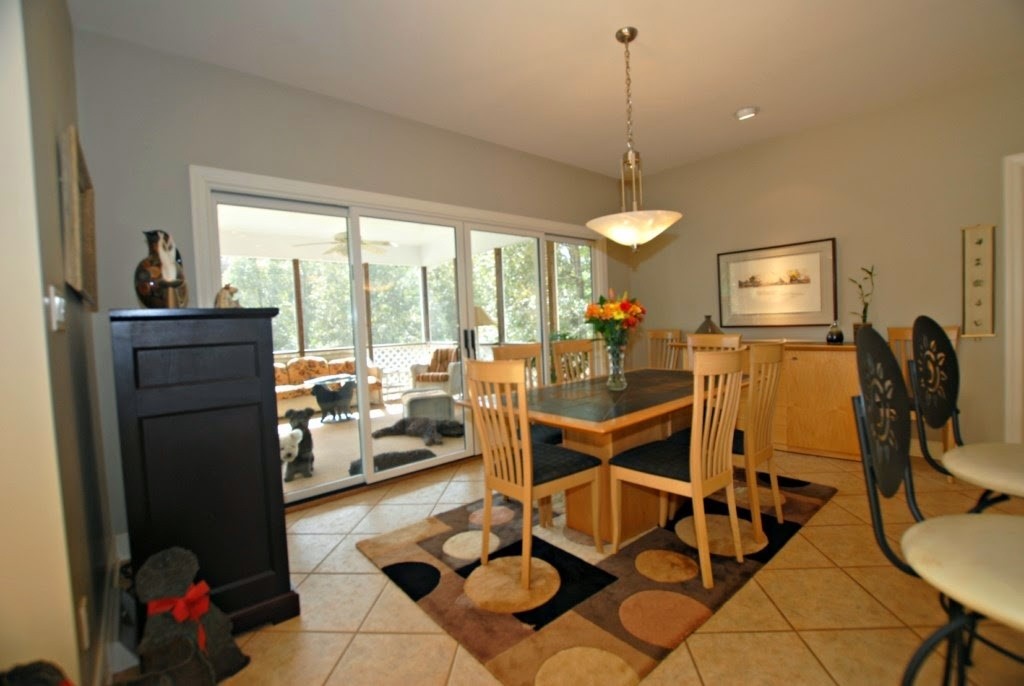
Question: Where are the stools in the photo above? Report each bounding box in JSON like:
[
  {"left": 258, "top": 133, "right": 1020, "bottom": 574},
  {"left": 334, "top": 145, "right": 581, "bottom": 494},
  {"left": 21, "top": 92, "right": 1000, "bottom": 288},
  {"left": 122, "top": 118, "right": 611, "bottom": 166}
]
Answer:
[
  {"left": 908, "top": 313, "right": 1024, "bottom": 663},
  {"left": 849, "top": 322, "right": 1024, "bottom": 686},
  {"left": 402, "top": 390, "right": 455, "bottom": 426}
]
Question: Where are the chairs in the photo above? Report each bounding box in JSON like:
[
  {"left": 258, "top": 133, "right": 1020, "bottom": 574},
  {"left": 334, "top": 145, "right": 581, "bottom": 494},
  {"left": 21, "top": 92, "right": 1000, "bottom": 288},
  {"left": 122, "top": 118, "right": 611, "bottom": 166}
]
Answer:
[
  {"left": 886, "top": 324, "right": 960, "bottom": 480},
  {"left": 667, "top": 339, "right": 790, "bottom": 541},
  {"left": 464, "top": 360, "right": 601, "bottom": 588},
  {"left": 491, "top": 344, "right": 545, "bottom": 395},
  {"left": 553, "top": 340, "right": 594, "bottom": 383},
  {"left": 670, "top": 330, "right": 743, "bottom": 444},
  {"left": 647, "top": 328, "right": 680, "bottom": 371},
  {"left": 609, "top": 349, "right": 751, "bottom": 589}
]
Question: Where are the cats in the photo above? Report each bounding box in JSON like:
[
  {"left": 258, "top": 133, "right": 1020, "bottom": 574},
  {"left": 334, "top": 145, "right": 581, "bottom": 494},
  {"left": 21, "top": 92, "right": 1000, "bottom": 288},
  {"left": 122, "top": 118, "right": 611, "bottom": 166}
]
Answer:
[{"left": 348, "top": 449, "right": 438, "bottom": 476}]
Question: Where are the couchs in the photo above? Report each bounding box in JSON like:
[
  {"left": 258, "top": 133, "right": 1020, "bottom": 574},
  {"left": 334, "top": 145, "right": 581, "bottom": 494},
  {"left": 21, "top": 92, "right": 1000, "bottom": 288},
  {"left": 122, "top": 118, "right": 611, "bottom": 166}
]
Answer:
[
  {"left": 411, "top": 347, "right": 467, "bottom": 394},
  {"left": 275, "top": 355, "right": 385, "bottom": 423}
]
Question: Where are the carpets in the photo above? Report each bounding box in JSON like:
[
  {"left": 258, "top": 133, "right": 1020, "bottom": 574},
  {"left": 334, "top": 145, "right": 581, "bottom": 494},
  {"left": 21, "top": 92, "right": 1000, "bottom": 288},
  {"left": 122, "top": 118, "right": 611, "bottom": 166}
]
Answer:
[{"left": 354, "top": 463, "right": 838, "bottom": 686}]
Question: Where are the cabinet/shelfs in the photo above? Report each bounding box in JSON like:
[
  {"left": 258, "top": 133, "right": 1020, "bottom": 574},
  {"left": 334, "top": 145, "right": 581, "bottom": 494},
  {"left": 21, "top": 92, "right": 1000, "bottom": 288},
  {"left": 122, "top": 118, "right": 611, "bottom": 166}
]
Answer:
[
  {"left": 61, "top": 126, "right": 102, "bottom": 315},
  {"left": 112, "top": 304, "right": 298, "bottom": 635}
]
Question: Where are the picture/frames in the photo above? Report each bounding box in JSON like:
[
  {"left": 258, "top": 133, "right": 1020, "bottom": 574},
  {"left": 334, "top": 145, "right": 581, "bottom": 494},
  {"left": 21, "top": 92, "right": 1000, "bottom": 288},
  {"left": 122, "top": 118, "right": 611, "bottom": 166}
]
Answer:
[
  {"left": 961, "top": 224, "right": 996, "bottom": 339},
  {"left": 716, "top": 237, "right": 839, "bottom": 324}
]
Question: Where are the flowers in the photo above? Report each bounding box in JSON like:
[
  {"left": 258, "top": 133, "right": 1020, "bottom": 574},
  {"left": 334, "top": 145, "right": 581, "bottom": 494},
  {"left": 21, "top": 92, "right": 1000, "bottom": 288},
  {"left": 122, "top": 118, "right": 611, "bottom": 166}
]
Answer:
[{"left": 586, "top": 289, "right": 649, "bottom": 347}]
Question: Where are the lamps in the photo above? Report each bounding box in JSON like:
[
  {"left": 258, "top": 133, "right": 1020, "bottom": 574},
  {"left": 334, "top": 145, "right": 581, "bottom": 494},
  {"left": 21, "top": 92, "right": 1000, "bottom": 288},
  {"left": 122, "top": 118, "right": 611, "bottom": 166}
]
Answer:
[{"left": 584, "top": 29, "right": 682, "bottom": 246}]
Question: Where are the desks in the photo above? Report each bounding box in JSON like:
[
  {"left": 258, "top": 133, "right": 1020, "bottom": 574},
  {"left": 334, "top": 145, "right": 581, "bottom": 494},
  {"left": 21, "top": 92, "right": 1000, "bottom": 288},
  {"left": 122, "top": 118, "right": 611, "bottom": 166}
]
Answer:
[
  {"left": 454, "top": 368, "right": 771, "bottom": 543},
  {"left": 305, "top": 373, "right": 360, "bottom": 424}
]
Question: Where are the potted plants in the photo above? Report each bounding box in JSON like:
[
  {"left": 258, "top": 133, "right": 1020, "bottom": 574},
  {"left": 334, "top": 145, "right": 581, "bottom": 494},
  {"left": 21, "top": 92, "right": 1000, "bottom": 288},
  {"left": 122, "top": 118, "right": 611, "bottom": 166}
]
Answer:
[{"left": 846, "top": 264, "right": 877, "bottom": 344}]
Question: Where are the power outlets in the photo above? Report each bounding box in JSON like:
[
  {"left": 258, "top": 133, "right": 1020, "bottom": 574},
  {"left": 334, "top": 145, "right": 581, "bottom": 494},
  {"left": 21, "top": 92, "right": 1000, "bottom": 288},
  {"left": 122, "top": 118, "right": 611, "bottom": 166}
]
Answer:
[{"left": 80, "top": 595, "right": 94, "bottom": 650}]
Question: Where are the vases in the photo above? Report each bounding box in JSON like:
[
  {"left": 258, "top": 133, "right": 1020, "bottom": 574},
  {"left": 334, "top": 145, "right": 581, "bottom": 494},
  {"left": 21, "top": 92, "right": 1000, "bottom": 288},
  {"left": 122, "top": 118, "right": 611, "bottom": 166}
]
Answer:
[{"left": 606, "top": 342, "right": 627, "bottom": 391}]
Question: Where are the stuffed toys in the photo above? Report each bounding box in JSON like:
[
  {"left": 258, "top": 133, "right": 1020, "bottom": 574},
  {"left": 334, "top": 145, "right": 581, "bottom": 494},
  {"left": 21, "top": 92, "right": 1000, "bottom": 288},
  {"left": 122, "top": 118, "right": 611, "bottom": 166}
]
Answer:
[{"left": 133, "top": 547, "right": 250, "bottom": 686}]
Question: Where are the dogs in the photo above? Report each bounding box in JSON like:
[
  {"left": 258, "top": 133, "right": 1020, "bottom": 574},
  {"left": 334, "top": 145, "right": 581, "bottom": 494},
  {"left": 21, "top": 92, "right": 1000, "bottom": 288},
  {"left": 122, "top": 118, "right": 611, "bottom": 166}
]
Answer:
[
  {"left": 280, "top": 408, "right": 314, "bottom": 483},
  {"left": 373, "top": 416, "right": 464, "bottom": 445},
  {"left": 310, "top": 381, "right": 356, "bottom": 422}
]
